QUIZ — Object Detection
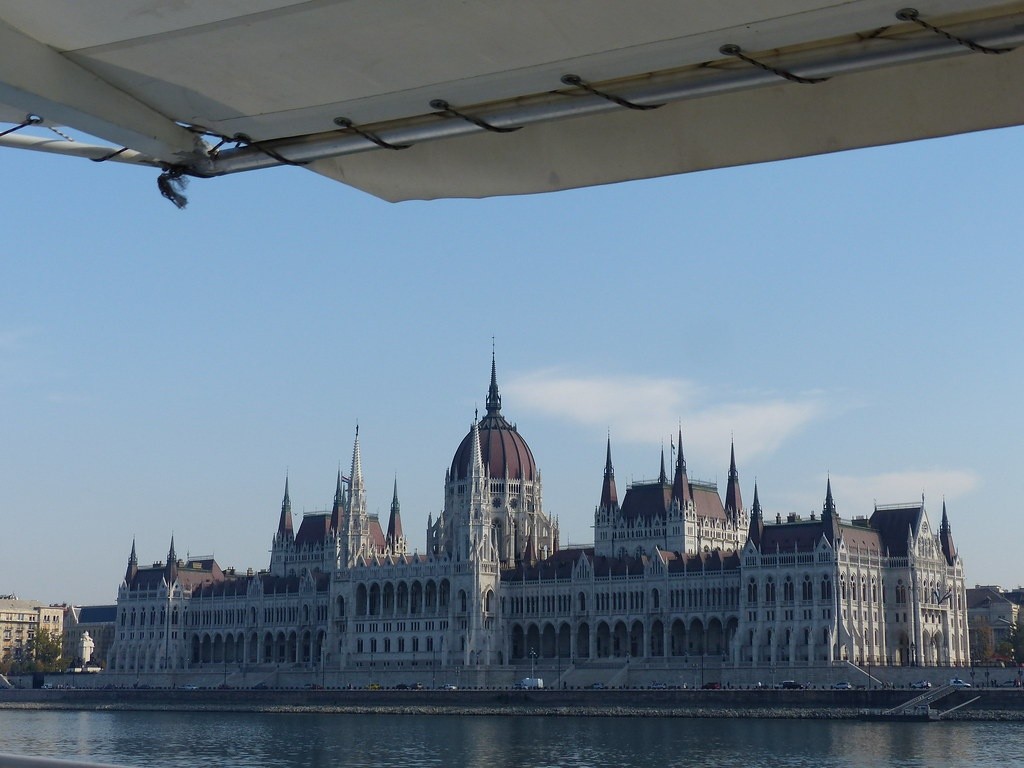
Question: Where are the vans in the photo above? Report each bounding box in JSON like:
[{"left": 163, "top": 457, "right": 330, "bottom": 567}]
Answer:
[
  {"left": 411, "top": 682, "right": 423, "bottom": 689},
  {"left": 949, "top": 679, "right": 971, "bottom": 688},
  {"left": 41, "top": 683, "right": 52, "bottom": 689},
  {"left": 680, "top": 683, "right": 688, "bottom": 689},
  {"left": 782, "top": 680, "right": 800, "bottom": 690}
]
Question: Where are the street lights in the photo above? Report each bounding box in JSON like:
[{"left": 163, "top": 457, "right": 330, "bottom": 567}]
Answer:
[{"left": 530, "top": 647, "right": 536, "bottom": 690}]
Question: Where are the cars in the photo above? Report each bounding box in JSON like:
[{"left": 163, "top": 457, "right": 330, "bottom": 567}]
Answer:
[
  {"left": 833, "top": 681, "right": 851, "bottom": 689},
  {"left": 438, "top": 683, "right": 457, "bottom": 690},
  {"left": 590, "top": 682, "right": 605, "bottom": 689},
  {"left": 910, "top": 680, "right": 932, "bottom": 689},
  {"left": 177, "top": 683, "right": 199, "bottom": 690},
  {"left": 393, "top": 683, "right": 410, "bottom": 689},
  {"left": 367, "top": 683, "right": 382, "bottom": 690},
  {"left": 304, "top": 684, "right": 321, "bottom": 690},
  {"left": 512, "top": 683, "right": 521, "bottom": 690},
  {"left": 651, "top": 682, "right": 666, "bottom": 688},
  {"left": 702, "top": 681, "right": 720, "bottom": 689}
]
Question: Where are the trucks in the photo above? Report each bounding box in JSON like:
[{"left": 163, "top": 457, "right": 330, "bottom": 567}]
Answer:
[{"left": 521, "top": 678, "right": 543, "bottom": 690}]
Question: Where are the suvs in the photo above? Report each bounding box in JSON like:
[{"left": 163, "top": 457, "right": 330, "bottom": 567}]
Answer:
[{"left": 1000, "top": 680, "right": 1020, "bottom": 687}]
